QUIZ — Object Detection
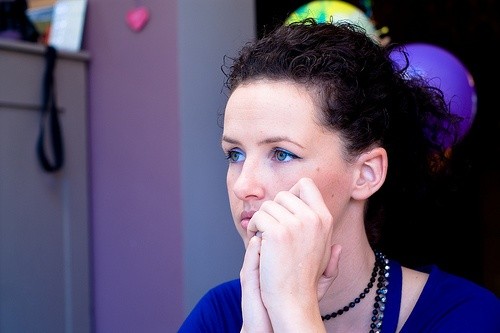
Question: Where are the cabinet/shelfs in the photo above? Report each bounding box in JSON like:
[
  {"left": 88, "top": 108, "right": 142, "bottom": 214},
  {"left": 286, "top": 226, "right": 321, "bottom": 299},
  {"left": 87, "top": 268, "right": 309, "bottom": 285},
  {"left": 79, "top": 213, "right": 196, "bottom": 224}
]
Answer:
[{"left": 0, "top": 40, "right": 92, "bottom": 333}]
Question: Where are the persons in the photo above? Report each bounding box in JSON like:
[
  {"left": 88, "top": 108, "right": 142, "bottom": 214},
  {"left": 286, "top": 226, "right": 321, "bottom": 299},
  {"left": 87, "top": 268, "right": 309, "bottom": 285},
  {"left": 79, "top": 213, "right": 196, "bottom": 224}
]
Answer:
[{"left": 175, "top": 18, "right": 500, "bottom": 333}]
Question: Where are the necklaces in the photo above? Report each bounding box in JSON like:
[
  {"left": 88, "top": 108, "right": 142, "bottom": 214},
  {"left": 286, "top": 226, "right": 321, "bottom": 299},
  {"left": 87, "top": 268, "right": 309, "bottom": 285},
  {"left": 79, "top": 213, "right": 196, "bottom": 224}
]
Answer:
[{"left": 320, "top": 248, "right": 390, "bottom": 333}]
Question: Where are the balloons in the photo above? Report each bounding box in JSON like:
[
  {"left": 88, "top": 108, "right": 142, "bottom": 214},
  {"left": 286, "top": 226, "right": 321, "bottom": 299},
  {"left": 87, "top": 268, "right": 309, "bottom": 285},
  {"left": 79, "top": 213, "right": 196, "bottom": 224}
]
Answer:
[{"left": 282, "top": 0, "right": 481, "bottom": 176}]
